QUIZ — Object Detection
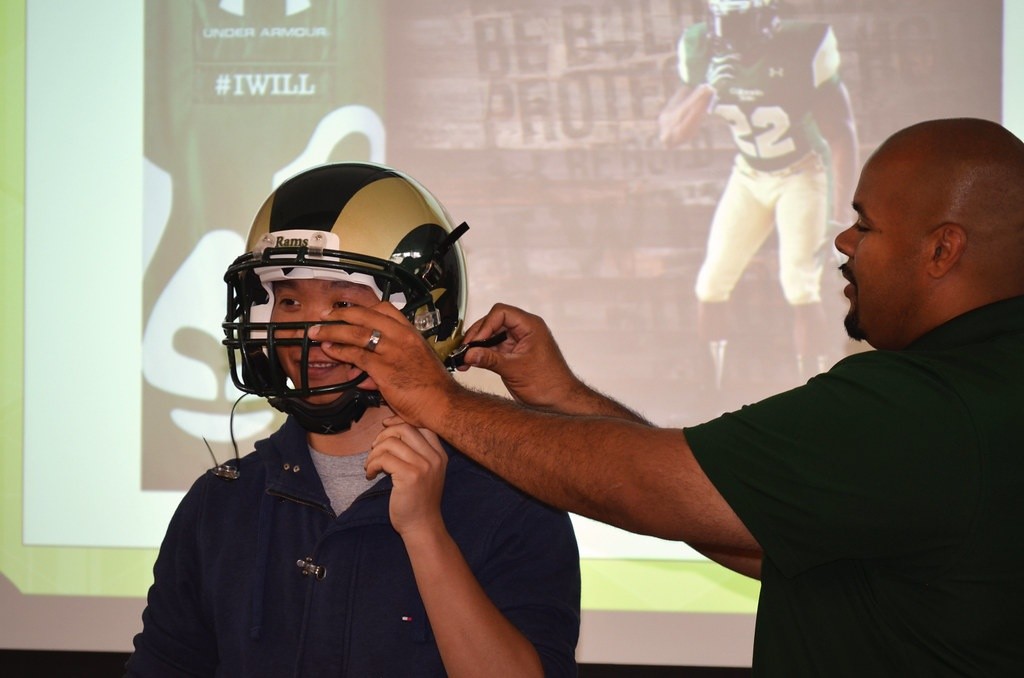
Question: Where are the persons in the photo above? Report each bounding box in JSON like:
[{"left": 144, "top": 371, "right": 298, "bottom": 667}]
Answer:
[
  {"left": 305, "top": 113, "right": 1024, "bottom": 676},
  {"left": 650, "top": 0, "right": 861, "bottom": 413},
  {"left": 120, "top": 164, "right": 581, "bottom": 676}
]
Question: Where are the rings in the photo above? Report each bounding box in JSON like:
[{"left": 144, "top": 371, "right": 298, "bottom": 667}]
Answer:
[{"left": 363, "top": 328, "right": 383, "bottom": 352}]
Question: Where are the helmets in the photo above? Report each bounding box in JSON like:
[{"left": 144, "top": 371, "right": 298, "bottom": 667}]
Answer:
[
  {"left": 223, "top": 162, "right": 507, "bottom": 435},
  {"left": 705, "top": 0, "right": 783, "bottom": 53}
]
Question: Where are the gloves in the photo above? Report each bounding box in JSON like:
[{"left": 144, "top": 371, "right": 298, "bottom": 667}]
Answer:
[{"left": 705, "top": 46, "right": 742, "bottom": 91}]
